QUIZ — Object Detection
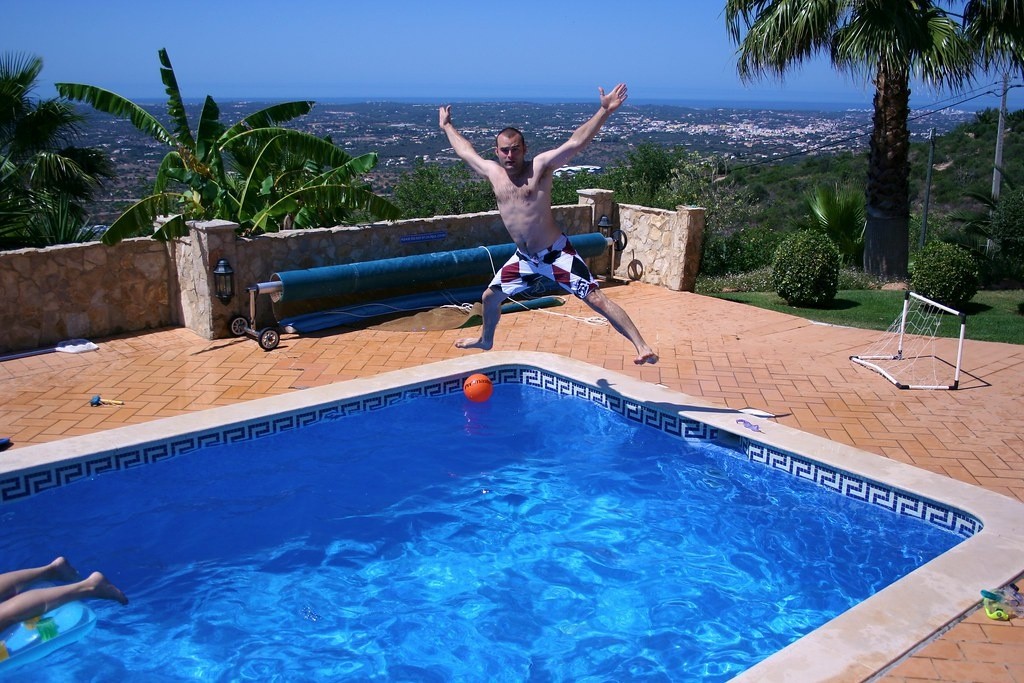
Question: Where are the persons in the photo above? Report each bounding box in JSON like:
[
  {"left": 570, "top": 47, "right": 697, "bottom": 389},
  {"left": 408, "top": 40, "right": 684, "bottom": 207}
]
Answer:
[
  {"left": 0, "top": 555, "right": 128, "bottom": 633},
  {"left": 438, "top": 81, "right": 661, "bottom": 365}
]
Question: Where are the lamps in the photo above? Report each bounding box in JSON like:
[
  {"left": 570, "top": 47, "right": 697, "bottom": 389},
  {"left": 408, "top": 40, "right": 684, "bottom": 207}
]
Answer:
[
  {"left": 212, "top": 259, "right": 236, "bottom": 306},
  {"left": 597, "top": 216, "right": 613, "bottom": 238}
]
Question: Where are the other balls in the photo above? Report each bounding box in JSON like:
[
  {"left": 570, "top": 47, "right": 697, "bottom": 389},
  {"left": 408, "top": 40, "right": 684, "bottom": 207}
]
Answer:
[{"left": 462, "top": 373, "right": 493, "bottom": 402}]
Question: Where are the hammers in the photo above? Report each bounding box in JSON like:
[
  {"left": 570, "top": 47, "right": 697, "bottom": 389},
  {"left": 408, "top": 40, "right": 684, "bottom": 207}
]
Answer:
[{"left": 89, "top": 394, "right": 125, "bottom": 407}]
